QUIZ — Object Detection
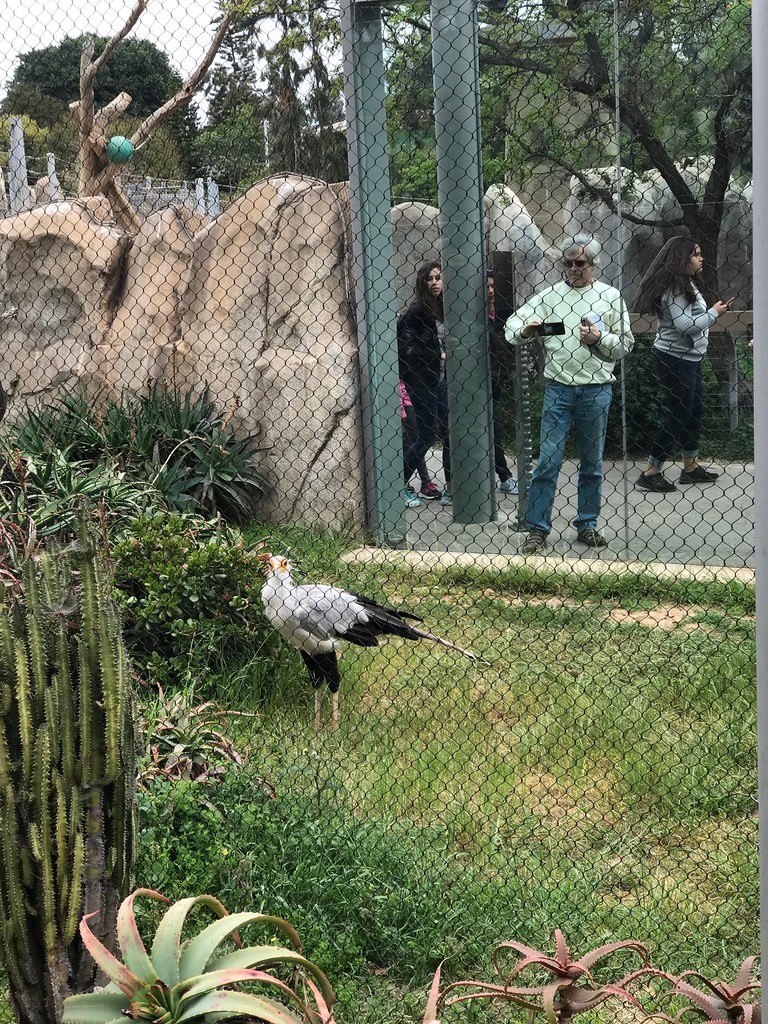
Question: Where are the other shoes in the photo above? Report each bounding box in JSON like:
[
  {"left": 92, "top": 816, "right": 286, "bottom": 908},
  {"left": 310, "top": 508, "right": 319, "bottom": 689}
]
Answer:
[{"left": 407, "top": 486, "right": 415, "bottom": 493}]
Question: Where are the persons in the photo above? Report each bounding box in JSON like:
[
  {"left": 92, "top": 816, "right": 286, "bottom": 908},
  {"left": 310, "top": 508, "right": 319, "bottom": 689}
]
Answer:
[
  {"left": 397, "top": 261, "right": 518, "bottom": 508},
  {"left": 633, "top": 237, "right": 729, "bottom": 492},
  {"left": 503, "top": 233, "right": 635, "bottom": 553}
]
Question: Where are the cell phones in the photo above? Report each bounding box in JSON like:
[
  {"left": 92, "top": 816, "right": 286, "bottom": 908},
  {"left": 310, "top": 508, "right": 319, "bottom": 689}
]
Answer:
[
  {"left": 726, "top": 296, "right": 735, "bottom": 306},
  {"left": 538, "top": 322, "right": 565, "bottom": 336}
]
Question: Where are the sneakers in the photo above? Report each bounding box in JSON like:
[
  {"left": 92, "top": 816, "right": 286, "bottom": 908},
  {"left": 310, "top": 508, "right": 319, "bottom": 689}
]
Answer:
[
  {"left": 405, "top": 494, "right": 419, "bottom": 507},
  {"left": 441, "top": 491, "right": 453, "bottom": 505},
  {"left": 636, "top": 471, "right": 677, "bottom": 492},
  {"left": 577, "top": 530, "right": 606, "bottom": 547},
  {"left": 418, "top": 482, "right": 442, "bottom": 499},
  {"left": 501, "top": 478, "right": 518, "bottom": 494},
  {"left": 523, "top": 532, "right": 546, "bottom": 553},
  {"left": 679, "top": 466, "right": 719, "bottom": 483}
]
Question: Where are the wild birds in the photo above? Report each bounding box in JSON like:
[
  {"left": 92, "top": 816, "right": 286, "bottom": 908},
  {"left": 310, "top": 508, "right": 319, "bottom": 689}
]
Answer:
[{"left": 260, "top": 555, "right": 490, "bottom": 735}]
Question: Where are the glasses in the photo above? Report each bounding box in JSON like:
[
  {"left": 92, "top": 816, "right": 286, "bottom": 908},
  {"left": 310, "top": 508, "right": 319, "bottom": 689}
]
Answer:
[{"left": 564, "top": 261, "right": 588, "bottom": 267}]
